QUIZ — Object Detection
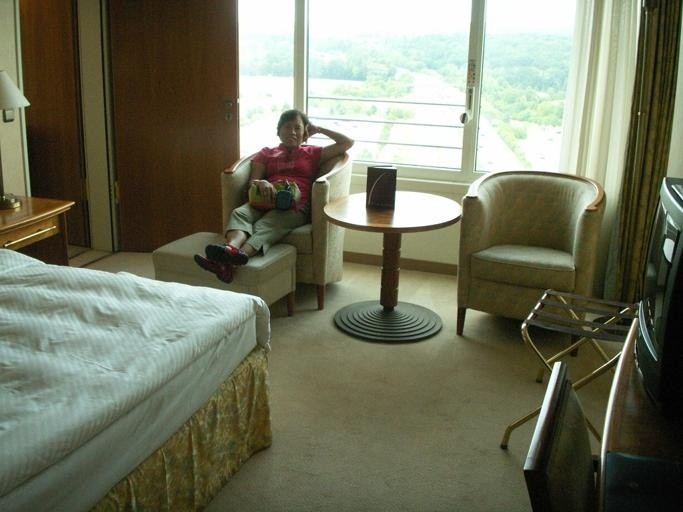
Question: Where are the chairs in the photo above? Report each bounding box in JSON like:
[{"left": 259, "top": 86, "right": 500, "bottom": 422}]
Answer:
[
  {"left": 524, "top": 359, "right": 595, "bottom": 512},
  {"left": 219, "top": 151, "right": 351, "bottom": 312},
  {"left": 457, "top": 168, "right": 606, "bottom": 358}
]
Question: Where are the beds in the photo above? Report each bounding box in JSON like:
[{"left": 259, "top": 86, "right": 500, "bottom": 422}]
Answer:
[{"left": 0, "top": 248, "right": 273, "bottom": 512}]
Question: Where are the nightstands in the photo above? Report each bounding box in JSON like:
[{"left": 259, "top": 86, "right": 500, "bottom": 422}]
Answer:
[{"left": 0, "top": 197, "right": 76, "bottom": 266}]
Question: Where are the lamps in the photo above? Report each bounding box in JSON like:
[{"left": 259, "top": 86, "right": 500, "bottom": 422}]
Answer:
[{"left": 1, "top": 70, "right": 31, "bottom": 211}]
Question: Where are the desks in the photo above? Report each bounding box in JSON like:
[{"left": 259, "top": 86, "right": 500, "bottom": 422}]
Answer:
[{"left": 323, "top": 190, "right": 463, "bottom": 344}]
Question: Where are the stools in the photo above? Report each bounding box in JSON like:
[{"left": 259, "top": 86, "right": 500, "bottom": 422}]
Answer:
[
  {"left": 151, "top": 231, "right": 298, "bottom": 320},
  {"left": 499, "top": 285, "right": 646, "bottom": 452}
]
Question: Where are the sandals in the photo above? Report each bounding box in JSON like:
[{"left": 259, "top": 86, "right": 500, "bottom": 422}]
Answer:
[{"left": 194, "top": 244, "right": 249, "bottom": 283}]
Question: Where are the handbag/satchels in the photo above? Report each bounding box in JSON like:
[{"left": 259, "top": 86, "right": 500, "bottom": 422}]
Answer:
[{"left": 248, "top": 179, "right": 301, "bottom": 210}]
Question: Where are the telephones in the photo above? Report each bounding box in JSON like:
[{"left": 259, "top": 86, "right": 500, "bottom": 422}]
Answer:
[{"left": 0, "top": 193, "right": 22, "bottom": 209}]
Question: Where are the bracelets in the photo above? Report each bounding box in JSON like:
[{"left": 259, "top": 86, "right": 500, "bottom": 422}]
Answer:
[
  {"left": 251, "top": 180, "right": 258, "bottom": 187},
  {"left": 319, "top": 127, "right": 320, "bottom": 134}
]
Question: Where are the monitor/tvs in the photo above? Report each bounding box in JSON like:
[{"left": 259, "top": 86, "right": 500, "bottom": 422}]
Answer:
[{"left": 635, "top": 176, "right": 683, "bottom": 415}]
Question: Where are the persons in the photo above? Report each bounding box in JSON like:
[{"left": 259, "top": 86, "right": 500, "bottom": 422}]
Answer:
[{"left": 194, "top": 110, "right": 354, "bottom": 285}]
